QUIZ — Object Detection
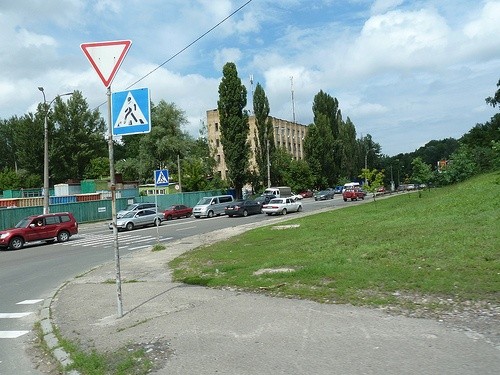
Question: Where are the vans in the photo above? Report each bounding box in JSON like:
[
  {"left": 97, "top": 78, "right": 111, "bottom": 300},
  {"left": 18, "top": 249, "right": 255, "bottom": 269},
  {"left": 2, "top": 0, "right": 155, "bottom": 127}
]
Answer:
[
  {"left": 324, "top": 182, "right": 360, "bottom": 194},
  {"left": 192, "top": 194, "right": 235, "bottom": 219}
]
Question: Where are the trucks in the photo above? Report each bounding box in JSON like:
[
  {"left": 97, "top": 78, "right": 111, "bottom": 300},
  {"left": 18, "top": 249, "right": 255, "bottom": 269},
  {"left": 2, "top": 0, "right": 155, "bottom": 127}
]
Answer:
[{"left": 261, "top": 186, "right": 291, "bottom": 198}]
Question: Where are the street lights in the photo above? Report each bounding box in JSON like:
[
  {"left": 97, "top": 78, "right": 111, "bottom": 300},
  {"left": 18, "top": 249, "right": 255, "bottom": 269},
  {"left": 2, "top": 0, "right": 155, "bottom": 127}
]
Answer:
[
  {"left": 36, "top": 86, "right": 74, "bottom": 214},
  {"left": 391, "top": 159, "right": 399, "bottom": 182},
  {"left": 364, "top": 148, "right": 377, "bottom": 186},
  {"left": 266, "top": 125, "right": 279, "bottom": 188},
  {"left": 398, "top": 166, "right": 405, "bottom": 183}
]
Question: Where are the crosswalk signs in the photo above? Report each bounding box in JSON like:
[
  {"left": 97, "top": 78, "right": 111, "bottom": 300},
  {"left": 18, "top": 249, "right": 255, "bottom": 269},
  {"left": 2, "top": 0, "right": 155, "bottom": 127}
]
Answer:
[
  {"left": 155, "top": 169, "right": 170, "bottom": 186},
  {"left": 111, "top": 87, "right": 152, "bottom": 136}
]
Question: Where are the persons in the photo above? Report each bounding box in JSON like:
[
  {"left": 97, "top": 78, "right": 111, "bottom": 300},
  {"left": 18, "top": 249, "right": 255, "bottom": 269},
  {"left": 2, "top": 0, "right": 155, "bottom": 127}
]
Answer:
[{"left": 37, "top": 220, "right": 43, "bottom": 227}]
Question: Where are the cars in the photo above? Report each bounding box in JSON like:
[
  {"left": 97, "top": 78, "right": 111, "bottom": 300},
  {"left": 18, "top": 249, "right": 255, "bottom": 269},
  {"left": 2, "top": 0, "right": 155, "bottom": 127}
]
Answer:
[
  {"left": 224, "top": 199, "right": 263, "bottom": 217},
  {"left": 291, "top": 191, "right": 304, "bottom": 201},
  {"left": 299, "top": 190, "right": 313, "bottom": 198},
  {"left": 255, "top": 194, "right": 277, "bottom": 205},
  {"left": 262, "top": 197, "right": 303, "bottom": 216},
  {"left": 314, "top": 189, "right": 336, "bottom": 201},
  {"left": 108, "top": 209, "right": 165, "bottom": 232},
  {"left": 377, "top": 186, "right": 387, "bottom": 192},
  {"left": 162, "top": 204, "right": 193, "bottom": 221},
  {"left": 397, "top": 181, "right": 434, "bottom": 191}
]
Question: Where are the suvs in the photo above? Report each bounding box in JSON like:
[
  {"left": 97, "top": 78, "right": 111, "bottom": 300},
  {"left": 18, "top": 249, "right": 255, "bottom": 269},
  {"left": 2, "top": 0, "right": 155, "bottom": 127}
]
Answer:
[
  {"left": 0, "top": 212, "right": 78, "bottom": 251},
  {"left": 116, "top": 203, "right": 160, "bottom": 219},
  {"left": 342, "top": 187, "right": 365, "bottom": 201}
]
